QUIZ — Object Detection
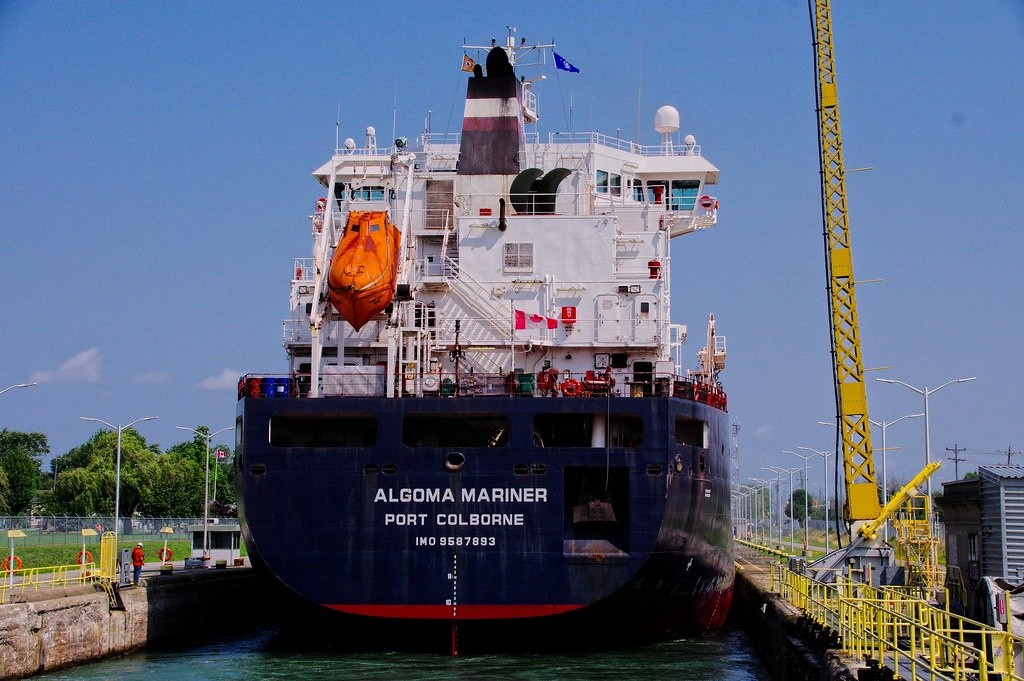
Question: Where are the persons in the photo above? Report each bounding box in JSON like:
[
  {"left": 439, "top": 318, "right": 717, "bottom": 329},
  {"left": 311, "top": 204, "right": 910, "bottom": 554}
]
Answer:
[{"left": 131, "top": 542, "right": 145, "bottom": 586}]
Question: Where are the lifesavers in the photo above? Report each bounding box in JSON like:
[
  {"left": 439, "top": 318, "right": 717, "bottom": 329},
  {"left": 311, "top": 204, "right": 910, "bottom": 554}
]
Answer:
[
  {"left": 563, "top": 378, "right": 581, "bottom": 397},
  {"left": 698, "top": 195, "right": 715, "bottom": 211},
  {"left": 76, "top": 551, "right": 93, "bottom": 566},
  {"left": 158, "top": 547, "right": 172, "bottom": 562},
  {"left": 296, "top": 268, "right": 303, "bottom": 280},
  {"left": 2, "top": 555, "right": 22, "bottom": 574}
]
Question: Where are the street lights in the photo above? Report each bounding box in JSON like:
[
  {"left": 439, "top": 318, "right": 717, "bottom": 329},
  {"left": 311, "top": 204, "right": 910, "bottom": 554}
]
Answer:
[
  {"left": 175, "top": 423, "right": 234, "bottom": 561},
  {"left": 725, "top": 372, "right": 979, "bottom": 567},
  {"left": 80, "top": 412, "right": 163, "bottom": 536}
]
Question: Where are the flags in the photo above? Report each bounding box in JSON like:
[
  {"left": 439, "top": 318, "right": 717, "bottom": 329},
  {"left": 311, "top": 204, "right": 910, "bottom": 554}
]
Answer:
[
  {"left": 553, "top": 51, "right": 581, "bottom": 74},
  {"left": 515, "top": 308, "right": 557, "bottom": 331},
  {"left": 462, "top": 54, "right": 477, "bottom": 72},
  {"left": 218, "top": 450, "right": 226, "bottom": 459}
]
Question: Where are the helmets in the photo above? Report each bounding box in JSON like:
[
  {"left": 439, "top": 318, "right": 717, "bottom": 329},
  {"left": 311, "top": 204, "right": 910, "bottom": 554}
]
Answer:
[{"left": 136, "top": 542, "right": 143, "bottom": 547}]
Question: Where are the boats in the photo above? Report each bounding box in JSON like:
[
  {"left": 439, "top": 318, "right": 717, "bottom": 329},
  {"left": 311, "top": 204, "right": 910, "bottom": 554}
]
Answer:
[{"left": 219, "top": 25, "right": 737, "bottom": 632}]
